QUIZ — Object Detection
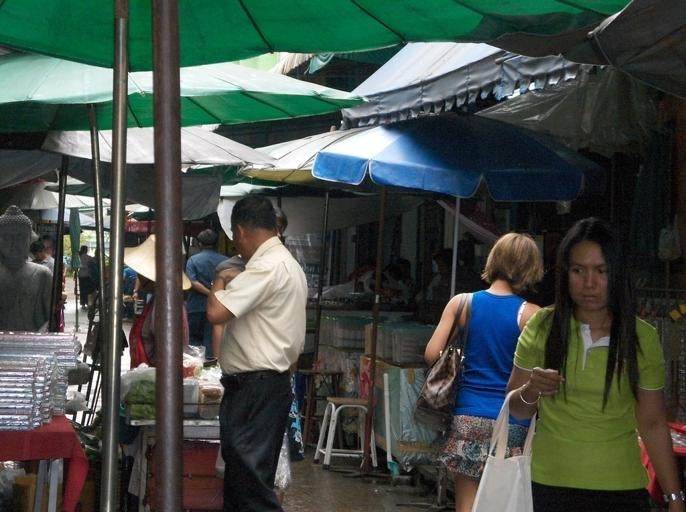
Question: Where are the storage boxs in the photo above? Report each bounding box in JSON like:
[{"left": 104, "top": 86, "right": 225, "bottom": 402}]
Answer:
[
  {"left": 142, "top": 439, "right": 224, "bottom": 477},
  {"left": 144, "top": 471, "right": 228, "bottom": 512}
]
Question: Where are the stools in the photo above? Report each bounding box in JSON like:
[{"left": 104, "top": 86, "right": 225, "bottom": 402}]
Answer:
[
  {"left": 297, "top": 367, "right": 350, "bottom": 456},
  {"left": 309, "top": 395, "right": 383, "bottom": 474}
]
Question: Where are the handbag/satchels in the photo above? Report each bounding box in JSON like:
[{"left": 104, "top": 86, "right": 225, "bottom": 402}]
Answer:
[
  {"left": 414, "top": 348, "right": 462, "bottom": 431},
  {"left": 472, "top": 455, "right": 533, "bottom": 512}
]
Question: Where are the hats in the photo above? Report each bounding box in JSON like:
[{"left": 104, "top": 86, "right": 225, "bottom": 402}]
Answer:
[
  {"left": 123, "top": 234, "right": 192, "bottom": 291},
  {"left": 197, "top": 229, "right": 217, "bottom": 244}
]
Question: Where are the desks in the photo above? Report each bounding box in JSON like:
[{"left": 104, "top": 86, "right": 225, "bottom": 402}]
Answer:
[
  {"left": 0, "top": 409, "right": 92, "bottom": 512},
  {"left": 310, "top": 343, "right": 365, "bottom": 451},
  {"left": 353, "top": 349, "right": 448, "bottom": 486},
  {"left": 118, "top": 408, "right": 292, "bottom": 511}
]
